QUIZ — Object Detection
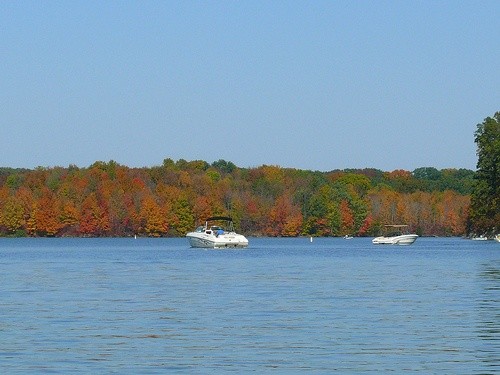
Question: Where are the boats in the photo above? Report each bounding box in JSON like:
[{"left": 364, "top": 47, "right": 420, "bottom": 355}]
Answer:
[
  {"left": 343, "top": 234, "right": 354, "bottom": 240},
  {"left": 186, "top": 216, "right": 248, "bottom": 249},
  {"left": 470, "top": 234, "right": 488, "bottom": 240},
  {"left": 372, "top": 225, "right": 420, "bottom": 245}
]
